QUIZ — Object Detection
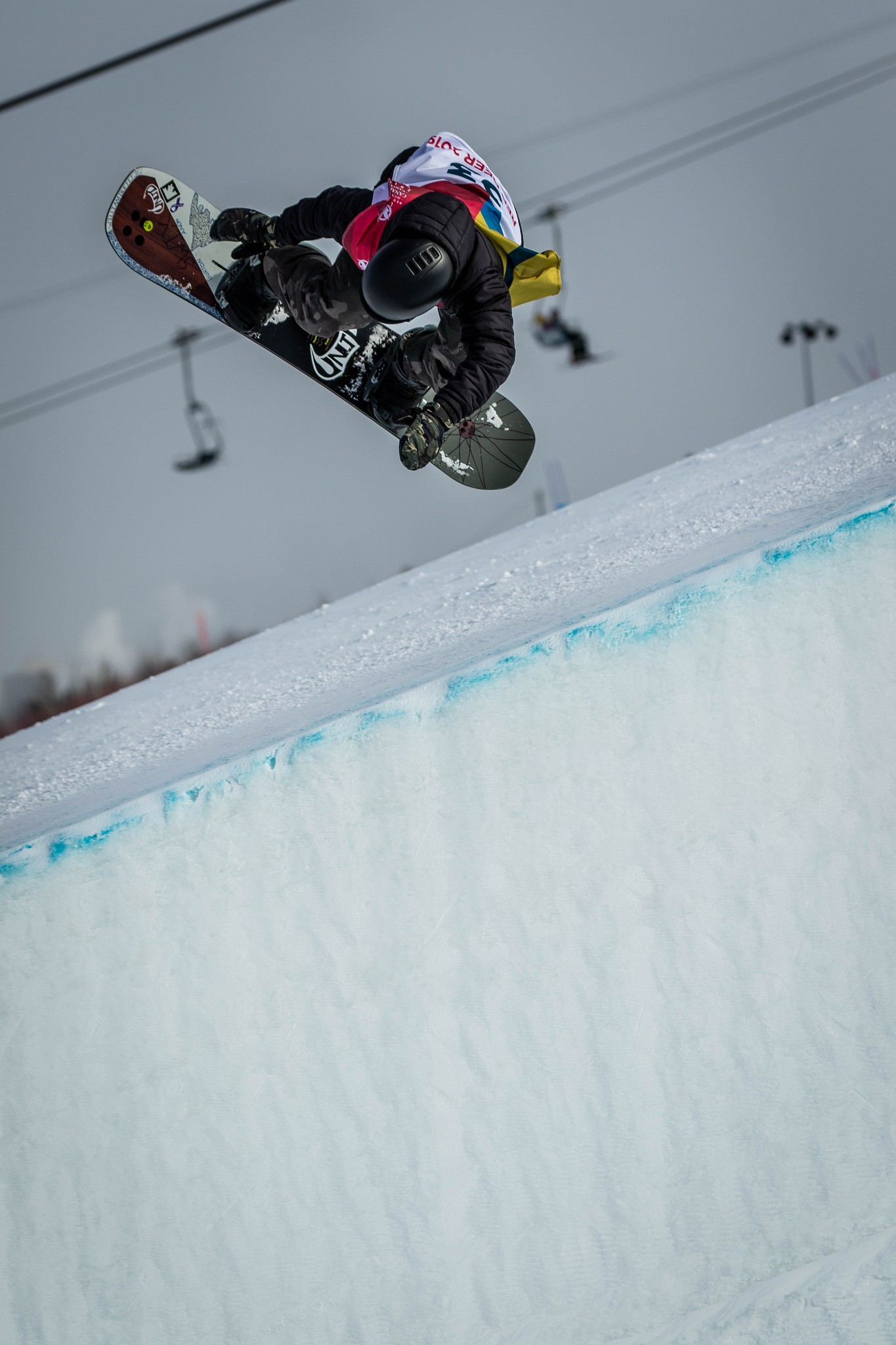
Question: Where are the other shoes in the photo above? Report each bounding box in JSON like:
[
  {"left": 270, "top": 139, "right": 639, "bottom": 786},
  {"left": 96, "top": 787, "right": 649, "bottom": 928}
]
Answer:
[
  {"left": 371, "top": 326, "right": 430, "bottom": 425},
  {"left": 234, "top": 270, "right": 275, "bottom": 327}
]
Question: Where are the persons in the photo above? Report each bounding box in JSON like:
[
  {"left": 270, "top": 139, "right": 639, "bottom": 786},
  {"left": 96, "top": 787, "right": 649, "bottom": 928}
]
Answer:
[
  {"left": 545, "top": 307, "right": 592, "bottom": 364},
  {"left": 215, "top": 132, "right": 561, "bottom": 471}
]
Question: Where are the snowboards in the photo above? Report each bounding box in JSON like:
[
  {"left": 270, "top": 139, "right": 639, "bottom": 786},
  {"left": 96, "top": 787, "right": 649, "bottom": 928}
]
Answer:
[{"left": 105, "top": 166, "right": 537, "bottom": 490}]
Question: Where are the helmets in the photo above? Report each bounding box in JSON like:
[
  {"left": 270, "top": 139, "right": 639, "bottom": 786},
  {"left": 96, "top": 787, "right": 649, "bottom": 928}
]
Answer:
[{"left": 361, "top": 234, "right": 453, "bottom": 322}]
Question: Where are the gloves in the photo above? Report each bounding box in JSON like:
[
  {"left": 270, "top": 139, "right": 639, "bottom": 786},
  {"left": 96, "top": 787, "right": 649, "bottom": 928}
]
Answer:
[
  {"left": 209, "top": 208, "right": 280, "bottom": 259},
  {"left": 392, "top": 402, "right": 453, "bottom": 470}
]
